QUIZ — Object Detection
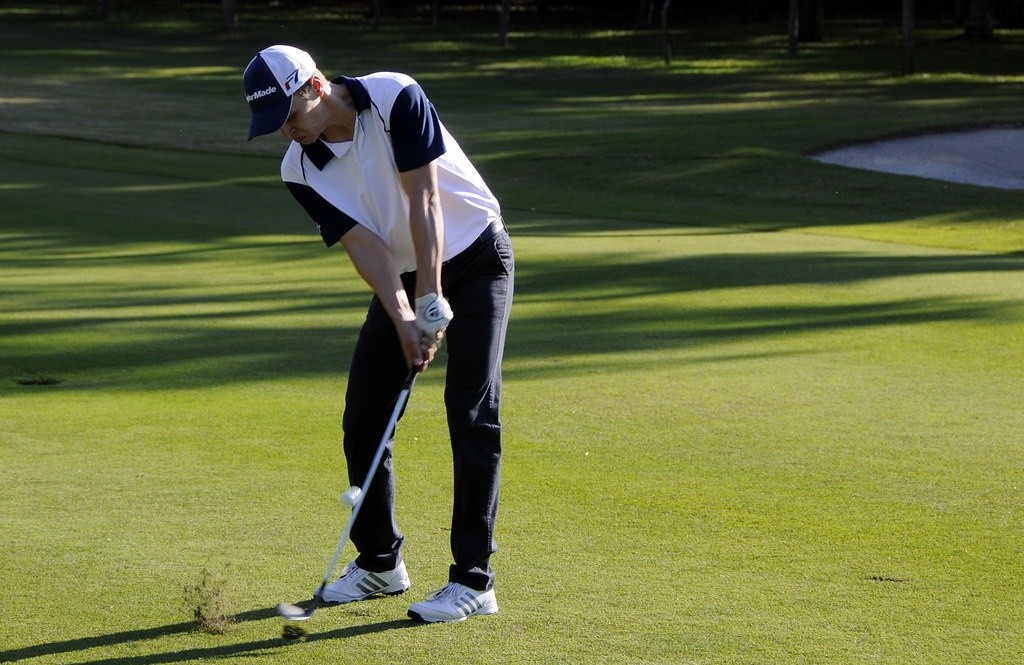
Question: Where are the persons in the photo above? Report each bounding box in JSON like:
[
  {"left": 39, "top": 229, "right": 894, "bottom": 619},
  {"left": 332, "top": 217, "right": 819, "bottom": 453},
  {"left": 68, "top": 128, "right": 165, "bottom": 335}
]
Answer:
[{"left": 241, "top": 44, "right": 515, "bottom": 622}]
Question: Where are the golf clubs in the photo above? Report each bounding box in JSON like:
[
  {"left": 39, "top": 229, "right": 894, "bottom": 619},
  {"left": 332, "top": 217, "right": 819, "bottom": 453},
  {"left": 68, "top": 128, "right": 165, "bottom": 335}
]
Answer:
[{"left": 277, "top": 321, "right": 439, "bottom": 623}]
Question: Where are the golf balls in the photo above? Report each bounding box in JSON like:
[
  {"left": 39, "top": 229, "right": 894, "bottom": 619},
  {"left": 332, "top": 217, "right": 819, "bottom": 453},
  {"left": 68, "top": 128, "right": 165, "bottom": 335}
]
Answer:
[{"left": 341, "top": 486, "right": 362, "bottom": 507}]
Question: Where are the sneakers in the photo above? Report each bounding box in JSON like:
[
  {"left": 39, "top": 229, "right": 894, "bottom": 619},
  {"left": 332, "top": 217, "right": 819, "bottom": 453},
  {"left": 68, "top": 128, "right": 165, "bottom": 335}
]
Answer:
[
  {"left": 407, "top": 577, "right": 498, "bottom": 622},
  {"left": 317, "top": 559, "right": 410, "bottom": 603}
]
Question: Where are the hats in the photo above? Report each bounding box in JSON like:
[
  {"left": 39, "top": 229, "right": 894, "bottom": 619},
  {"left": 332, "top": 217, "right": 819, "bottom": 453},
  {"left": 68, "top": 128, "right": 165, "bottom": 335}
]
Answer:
[{"left": 241, "top": 43, "right": 317, "bottom": 143}]
